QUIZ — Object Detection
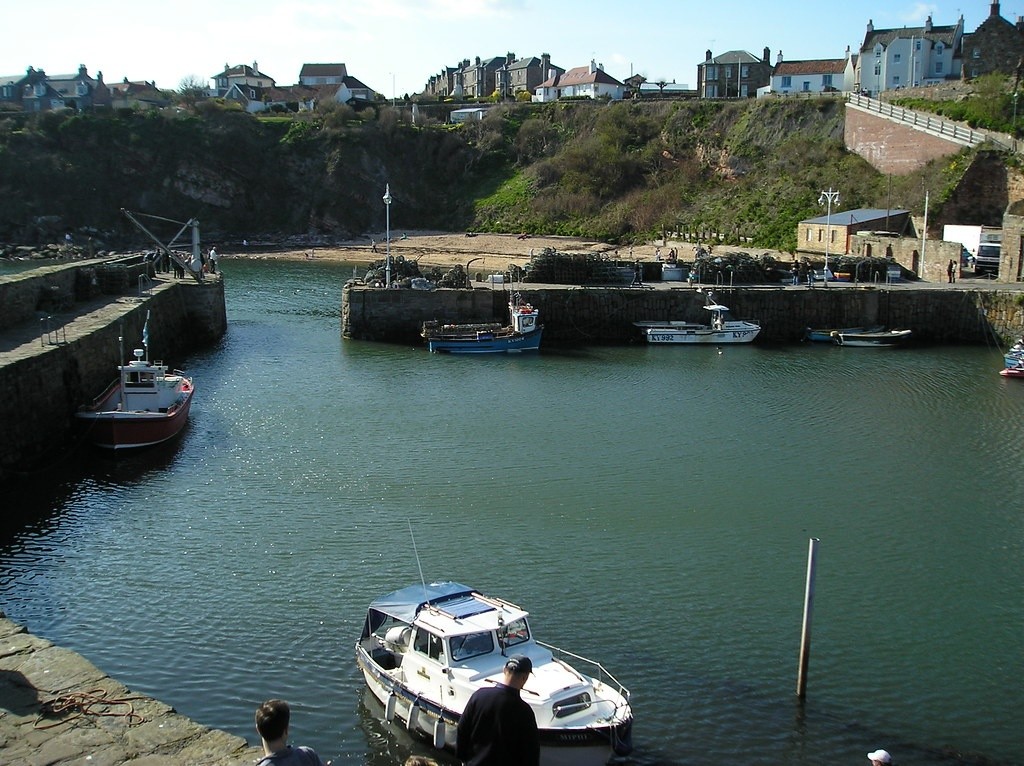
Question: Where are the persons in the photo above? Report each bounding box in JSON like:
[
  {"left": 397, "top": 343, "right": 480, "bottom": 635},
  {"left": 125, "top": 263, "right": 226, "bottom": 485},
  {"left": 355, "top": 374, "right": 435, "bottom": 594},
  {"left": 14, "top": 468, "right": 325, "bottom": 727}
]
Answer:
[
  {"left": 806, "top": 261, "right": 815, "bottom": 286},
  {"left": 371, "top": 238, "right": 377, "bottom": 252},
  {"left": 144, "top": 247, "right": 210, "bottom": 279},
  {"left": 669, "top": 247, "right": 678, "bottom": 259},
  {"left": 311, "top": 248, "right": 314, "bottom": 258},
  {"left": 455, "top": 653, "right": 540, "bottom": 766},
  {"left": 305, "top": 253, "right": 308, "bottom": 259},
  {"left": 947, "top": 259, "right": 957, "bottom": 283},
  {"left": 254, "top": 698, "right": 323, "bottom": 766},
  {"left": 790, "top": 260, "right": 802, "bottom": 285},
  {"left": 858, "top": 87, "right": 868, "bottom": 95},
  {"left": 400, "top": 233, "right": 406, "bottom": 240},
  {"left": 613, "top": 251, "right": 620, "bottom": 258},
  {"left": 867, "top": 749, "right": 892, "bottom": 766},
  {"left": 210, "top": 246, "right": 217, "bottom": 274},
  {"left": 656, "top": 247, "right": 661, "bottom": 261},
  {"left": 629, "top": 245, "right": 634, "bottom": 257},
  {"left": 895, "top": 85, "right": 900, "bottom": 91},
  {"left": 630, "top": 259, "right": 643, "bottom": 287}
]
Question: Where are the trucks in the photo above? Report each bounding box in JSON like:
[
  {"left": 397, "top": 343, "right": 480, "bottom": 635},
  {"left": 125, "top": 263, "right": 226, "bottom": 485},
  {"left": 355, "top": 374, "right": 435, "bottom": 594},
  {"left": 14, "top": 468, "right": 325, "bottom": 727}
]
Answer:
[{"left": 973, "top": 240, "right": 1002, "bottom": 278}]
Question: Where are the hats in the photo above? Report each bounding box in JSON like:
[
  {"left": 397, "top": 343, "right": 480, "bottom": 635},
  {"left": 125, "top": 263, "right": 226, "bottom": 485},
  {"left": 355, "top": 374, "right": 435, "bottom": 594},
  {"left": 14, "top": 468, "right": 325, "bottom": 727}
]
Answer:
[{"left": 505, "top": 654, "right": 534, "bottom": 675}]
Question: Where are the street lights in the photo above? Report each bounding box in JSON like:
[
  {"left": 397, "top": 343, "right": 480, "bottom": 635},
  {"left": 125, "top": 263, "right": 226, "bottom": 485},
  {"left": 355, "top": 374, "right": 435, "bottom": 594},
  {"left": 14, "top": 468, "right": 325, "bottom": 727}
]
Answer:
[
  {"left": 382, "top": 183, "right": 392, "bottom": 289},
  {"left": 817, "top": 187, "right": 841, "bottom": 288}
]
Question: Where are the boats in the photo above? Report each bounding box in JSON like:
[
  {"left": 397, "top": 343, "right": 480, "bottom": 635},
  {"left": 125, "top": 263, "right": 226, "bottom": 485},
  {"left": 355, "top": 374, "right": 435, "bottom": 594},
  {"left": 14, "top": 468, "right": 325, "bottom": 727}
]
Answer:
[
  {"left": 632, "top": 291, "right": 761, "bottom": 346},
  {"left": 353, "top": 518, "right": 634, "bottom": 766},
  {"left": 419, "top": 275, "right": 545, "bottom": 354},
  {"left": 998, "top": 338, "right": 1024, "bottom": 379},
  {"left": 804, "top": 326, "right": 913, "bottom": 347},
  {"left": 74, "top": 308, "right": 196, "bottom": 457}
]
